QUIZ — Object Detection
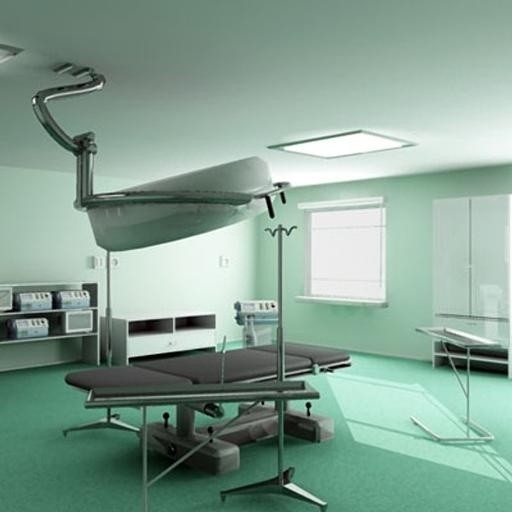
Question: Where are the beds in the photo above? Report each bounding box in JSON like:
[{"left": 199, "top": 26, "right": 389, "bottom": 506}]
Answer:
[{"left": 63, "top": 341, "right": 353, "bottom": 477}]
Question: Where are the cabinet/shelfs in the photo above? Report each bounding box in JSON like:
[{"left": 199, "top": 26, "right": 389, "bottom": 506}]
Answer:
[
  {"left": 0, "top": 281, "right": 101, "bottom": 368},
  {"left": 100, "top": 311, "right": 219, "bottom": 365}
]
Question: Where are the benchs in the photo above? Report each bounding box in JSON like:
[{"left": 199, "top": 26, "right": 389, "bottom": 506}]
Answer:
[{"left": 433, "top": 338, "right": 512, "bottom": 379}]
